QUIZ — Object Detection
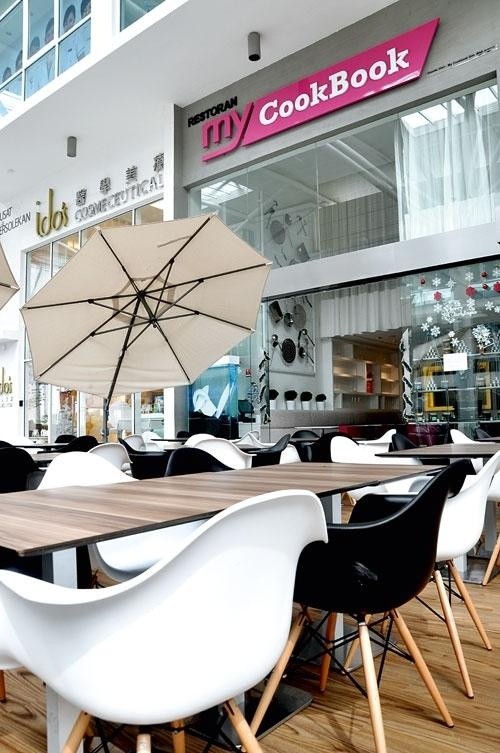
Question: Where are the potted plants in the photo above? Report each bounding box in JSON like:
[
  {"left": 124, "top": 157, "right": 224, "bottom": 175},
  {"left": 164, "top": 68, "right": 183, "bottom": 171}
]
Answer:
[
  {"left": 315, "top": 393, "right": 327, "bottom": 410},
  {"left": 300, "top": 391, "right": 313, "bottom": 409},
  {"left": 269, "top": 389, "right": 279, "bottom": 408},
  {"left": 284, "top": 389, "right": 298, "bottom": 409}
]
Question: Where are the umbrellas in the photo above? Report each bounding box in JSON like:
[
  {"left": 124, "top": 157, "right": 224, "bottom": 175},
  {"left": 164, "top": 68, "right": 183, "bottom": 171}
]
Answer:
[
  {"left": 20, "top": 210, "right": 276, "bottom": 447},
  {"left": 1, "top": 244, "right": 19, "bottom": 311}
]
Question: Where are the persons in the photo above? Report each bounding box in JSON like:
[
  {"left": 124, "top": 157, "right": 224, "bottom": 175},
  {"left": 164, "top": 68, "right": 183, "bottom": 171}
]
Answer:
[{"left": 2, "top": 1, "right": 91, "bottom": 108}]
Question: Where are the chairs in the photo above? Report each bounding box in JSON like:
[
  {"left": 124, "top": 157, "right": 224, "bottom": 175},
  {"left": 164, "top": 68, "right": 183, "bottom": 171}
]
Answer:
[{"left": 0, "top": 427, "right": 500, "bottom": 753}]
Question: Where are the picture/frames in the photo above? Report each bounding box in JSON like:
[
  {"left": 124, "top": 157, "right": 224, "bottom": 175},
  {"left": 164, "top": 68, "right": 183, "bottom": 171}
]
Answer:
[{"left": 424, "top": 359, "right": 492, "bottom": 410}]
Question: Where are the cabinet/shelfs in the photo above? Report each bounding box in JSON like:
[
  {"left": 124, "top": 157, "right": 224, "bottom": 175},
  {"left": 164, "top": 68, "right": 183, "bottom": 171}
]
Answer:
[{"left": 323, "top": 338, "right": 401, "bottom": 410}]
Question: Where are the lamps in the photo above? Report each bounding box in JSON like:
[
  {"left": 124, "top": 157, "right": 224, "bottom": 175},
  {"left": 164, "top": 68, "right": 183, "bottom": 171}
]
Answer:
[
  {"left": 248, "top": 30, "right": 262, "bottom": 61},
  {"left": 66, "top": 135, "right": 77, "bottom": 157}
]
